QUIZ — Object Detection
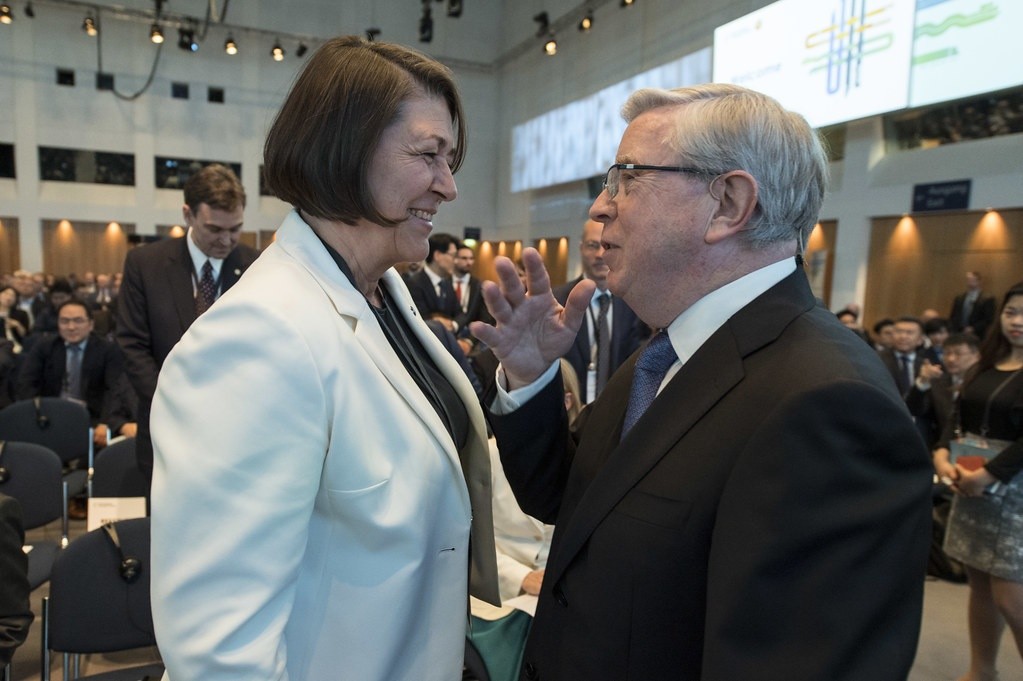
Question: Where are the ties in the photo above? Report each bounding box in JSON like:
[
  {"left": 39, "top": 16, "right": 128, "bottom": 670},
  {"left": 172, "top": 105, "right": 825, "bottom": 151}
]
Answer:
[
  {"left": 437, "top": 280, "right": 445, "bottom": 311},
  {"left": 196, "top": 259, "right": 216, "bottom": 317},
  {"left": 900, "top": 355, "right": 910, "bottom": 399},
  {"left": 595, "top": 293, "right": 610, "bottom": 400},
  {"left": 65, "top": 343, "right": 81, "bottom": 398},
  {"left": 617, "top": 329, "right": 675, "bottom": 442},
  {"left": 455, "top": 281, "right": 462, "bottom": 305}
]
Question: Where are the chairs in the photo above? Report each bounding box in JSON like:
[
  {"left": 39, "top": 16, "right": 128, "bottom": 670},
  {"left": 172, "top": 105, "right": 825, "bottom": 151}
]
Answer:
[
  {"left": 0, "top": 443, "right": 70, "bottom": 595},
  {"left": 86, "top": 428, "right": 152, "bottom": 512},
  {"left": 0, "top": 398, "right": 89, "bottom": 522},
  {"left": 43, "top": 518, "right": 162, "bottom": 681}
]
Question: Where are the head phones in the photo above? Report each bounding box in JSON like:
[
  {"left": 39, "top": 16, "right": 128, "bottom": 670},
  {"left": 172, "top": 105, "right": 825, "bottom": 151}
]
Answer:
[
  {"left": 34, "top": 395, "right": 50, "bottom": 429},
  {"left": 0, "top": 440, "right": 9, "bottom": 484},
  {"left": 101, "top": 521, "right": 142, "bottom": 584}
]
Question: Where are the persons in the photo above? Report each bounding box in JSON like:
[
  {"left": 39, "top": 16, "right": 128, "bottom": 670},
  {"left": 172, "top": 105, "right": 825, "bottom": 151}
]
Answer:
[{"left": 0, "top": 35, "right": 1023, "bottom": 681}]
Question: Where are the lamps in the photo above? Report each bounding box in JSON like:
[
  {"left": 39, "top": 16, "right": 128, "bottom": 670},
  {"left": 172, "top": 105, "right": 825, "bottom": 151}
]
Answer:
[
  {"left": 269, "top": 39, "right": 287, "bottom": 61},
  {"left": 149, "top": 20, "right": 164, "bottom": 43},
  {"left": 220, "top": 32, "right": 239, "bottom": 54},
  {"left": 0, "top": 5, "right": 15, "bottom": 24},
  {"left": 578, "top": 12, "right": 594, "bottom": 31},
  {"left": 542, "top": 37, "right": 557, "bottom": 54},
  {"left": 297, "top": 41, "right": 307, "bottom": 58},
  {"left": 81, "top": 15, "right": 97, "bottom": 35}
]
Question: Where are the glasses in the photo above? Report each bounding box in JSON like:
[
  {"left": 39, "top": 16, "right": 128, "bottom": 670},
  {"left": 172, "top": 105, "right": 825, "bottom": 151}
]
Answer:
[{"left": 599, "top": 164, "right": 721, "bottom": 201}]
